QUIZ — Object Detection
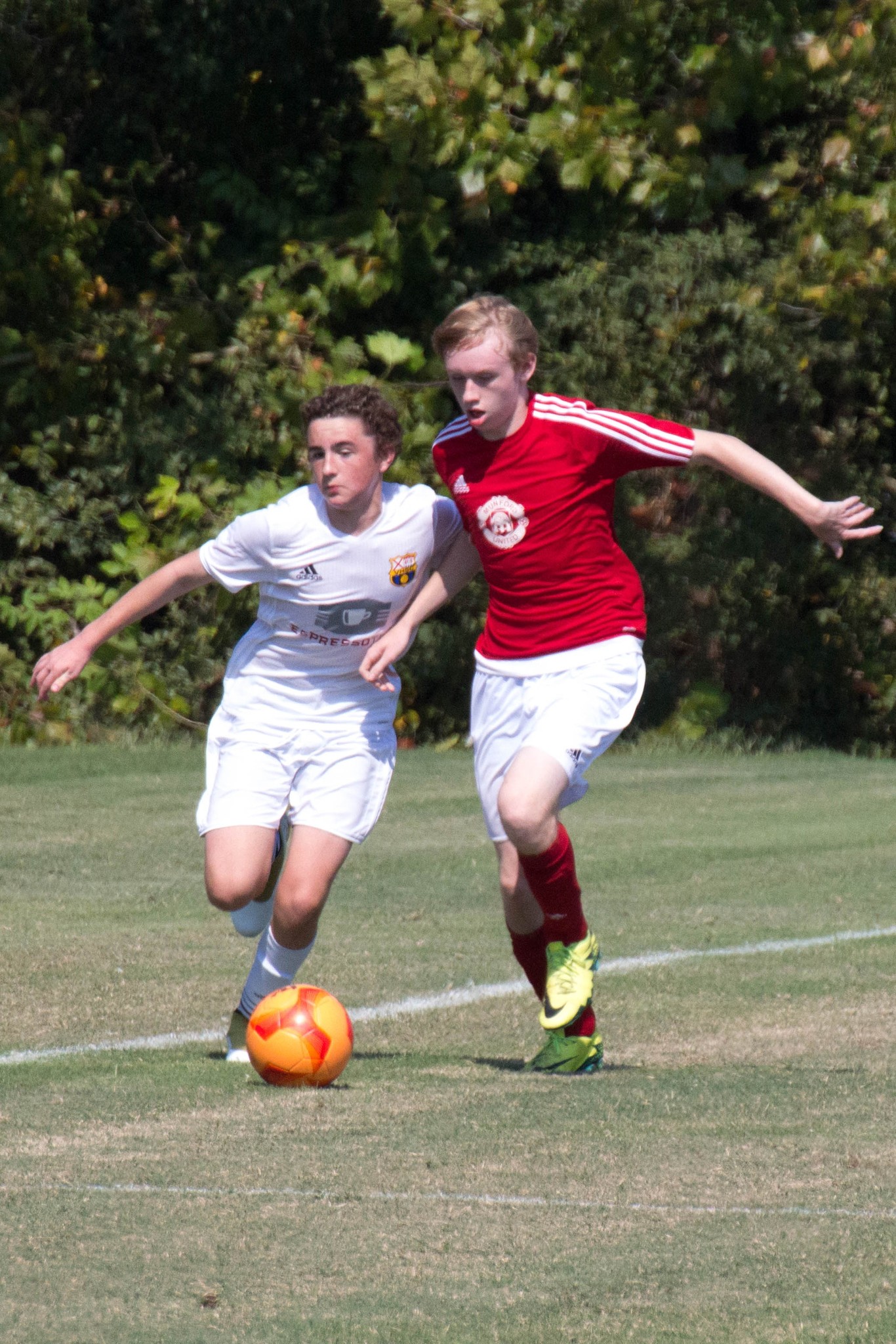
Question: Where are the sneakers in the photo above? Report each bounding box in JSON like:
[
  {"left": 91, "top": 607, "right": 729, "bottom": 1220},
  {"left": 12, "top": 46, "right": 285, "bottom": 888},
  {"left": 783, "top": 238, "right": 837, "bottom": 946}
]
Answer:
[
  {"left": 518, "top": 1029, "right": 603, "bottom": 1076},
  {"left": 227, "top": 1011, "right": 253, "bottom": 1064},
  {"left": 232, "top": 814, "right": 291, "bottom": 937},
  {"left": 538, "top": 929, "right": 603, "bottom": 1029}
]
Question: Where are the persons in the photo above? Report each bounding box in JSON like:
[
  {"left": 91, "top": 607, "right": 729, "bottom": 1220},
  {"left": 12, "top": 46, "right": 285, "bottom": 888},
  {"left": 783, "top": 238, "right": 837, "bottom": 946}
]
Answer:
[
  {"left": 360, "top": 294, "right": 882, "bottom": 1066},
  {"left": 30, "top": 384, "right": 465, "bottom": 1062}
]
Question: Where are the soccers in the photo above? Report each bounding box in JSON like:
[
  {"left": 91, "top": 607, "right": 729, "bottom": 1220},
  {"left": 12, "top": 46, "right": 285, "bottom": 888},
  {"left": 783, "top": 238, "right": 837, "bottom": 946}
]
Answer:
[{"left": 244, "top": 985, "right": 354, "bottom": 1090}]
